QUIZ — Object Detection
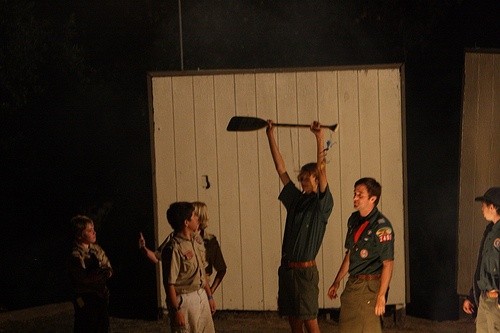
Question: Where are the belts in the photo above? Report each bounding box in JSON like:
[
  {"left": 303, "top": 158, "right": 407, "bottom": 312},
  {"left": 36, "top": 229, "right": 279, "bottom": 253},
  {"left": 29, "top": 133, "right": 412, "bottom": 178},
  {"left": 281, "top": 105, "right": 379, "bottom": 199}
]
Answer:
[
  {"left": 485, "top": 289, "right": 500, "bottom": 298},
  {"left": 281, "top": 256, "right": 316, "bottom": 268},
  {"left": 349, "top": 273, "right": 381, "bottom": 280}
]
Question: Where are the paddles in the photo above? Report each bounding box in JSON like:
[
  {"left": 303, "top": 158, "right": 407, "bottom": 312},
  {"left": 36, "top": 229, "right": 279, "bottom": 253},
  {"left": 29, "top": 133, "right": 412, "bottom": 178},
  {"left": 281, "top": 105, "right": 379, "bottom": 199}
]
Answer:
[{"left": 226, "top": 115, "right": 340, "bottom": 133}]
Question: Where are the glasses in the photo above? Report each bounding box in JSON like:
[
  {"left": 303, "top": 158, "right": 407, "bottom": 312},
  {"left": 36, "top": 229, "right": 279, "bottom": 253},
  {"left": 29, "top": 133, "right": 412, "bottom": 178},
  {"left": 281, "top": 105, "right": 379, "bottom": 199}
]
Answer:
[{"left": 298, "top": 175, "right": 310, "bottom": 182}]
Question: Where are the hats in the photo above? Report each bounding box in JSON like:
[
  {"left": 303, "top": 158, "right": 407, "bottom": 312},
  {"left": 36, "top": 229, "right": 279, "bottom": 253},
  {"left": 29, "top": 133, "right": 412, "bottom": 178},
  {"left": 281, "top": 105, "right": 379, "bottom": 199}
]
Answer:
[{"left": 474, "top": 187, "right": 500, "bottom": 205}]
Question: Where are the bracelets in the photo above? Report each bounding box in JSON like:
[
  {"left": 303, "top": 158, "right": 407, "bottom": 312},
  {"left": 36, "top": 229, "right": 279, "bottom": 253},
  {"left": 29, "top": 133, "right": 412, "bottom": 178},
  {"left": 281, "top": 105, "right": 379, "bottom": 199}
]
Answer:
[
  {"left": 171, "top": 307, "right": 181, "bottom": 313},
  {"left": 208, "top": 296, "right": 213, "bottom": 300}
]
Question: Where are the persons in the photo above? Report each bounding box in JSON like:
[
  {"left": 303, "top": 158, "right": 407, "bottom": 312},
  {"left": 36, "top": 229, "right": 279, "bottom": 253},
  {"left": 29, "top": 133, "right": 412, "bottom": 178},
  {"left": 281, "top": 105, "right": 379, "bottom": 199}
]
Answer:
[
  {"left": 265, "top": 119, "right": 334, "bottom": 333},
  {"left": 463, "top": 186, "right": 500, "bottom": 333},
  {"left": 65, "top": 214, "right": 113, "bottom": 333},
  {"left": 162, "top": 201, "right": 217, "bottom": 333},
  {"left": 328, "top": 177, "right": 395, "bottom": 333},
  {"left": 137, "top": 201, "right": 227, "bottom": 295}
]
